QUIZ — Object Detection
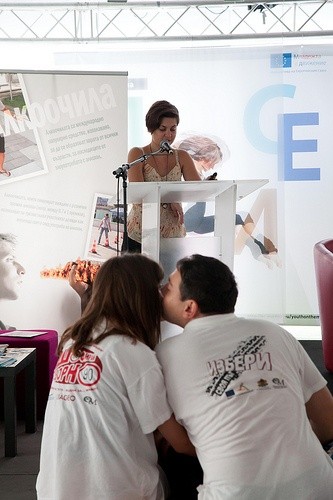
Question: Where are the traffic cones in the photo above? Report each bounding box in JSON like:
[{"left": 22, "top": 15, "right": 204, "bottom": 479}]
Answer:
[
  {"left": 89, "top": 239, "right": 97, "bottom": 254},
  {"left": 105, "top": 238, "right": 109, "bottom": 247}
]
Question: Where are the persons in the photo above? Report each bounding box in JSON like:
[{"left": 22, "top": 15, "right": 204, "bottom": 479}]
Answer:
[
  {"left": 179, "top": 135, "right": 278, "bottom": 262},
  {"left": 96, "top": 213, "right": 112, "bottom": 244},
  {"left": 36, "top": 254, "right": 197, "bottom": 500},
  {"left": 0, "top": 232, "right": 26, "bottom": 330},
  {"left": 161, "top": 253, "right": 333, "bottom": 500},
  {"left": 119, "top": 100, "right": 202, "bottom": 256},
  {"left": 0, "top": 100, "right": 12, "bottom": 176}
]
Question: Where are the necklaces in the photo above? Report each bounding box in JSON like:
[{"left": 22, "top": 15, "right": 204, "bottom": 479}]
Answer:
[{"left": 148, "top": 143, "right": 168, "bottom": 209}]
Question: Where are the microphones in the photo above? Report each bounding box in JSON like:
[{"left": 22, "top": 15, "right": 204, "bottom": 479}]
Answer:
[{"left": 161, "top": 139, "right": 174, "bottom": 154}]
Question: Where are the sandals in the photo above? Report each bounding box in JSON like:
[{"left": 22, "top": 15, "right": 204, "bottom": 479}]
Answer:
[{"left": 0, "top": 169, "right": 11, "bottom": 176}]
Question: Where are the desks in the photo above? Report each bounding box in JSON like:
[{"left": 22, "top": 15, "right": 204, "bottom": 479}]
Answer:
[
  {"left": 0, "top": 348, "right": 38, "bottom": 458},
  {"left": 0, "top": 329, "right": 58, "bottom": 423}
]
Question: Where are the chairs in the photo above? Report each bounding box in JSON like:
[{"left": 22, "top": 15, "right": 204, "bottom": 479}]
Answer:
[{"left": 314, "top": 238, "right": 333, "bottom": 373}]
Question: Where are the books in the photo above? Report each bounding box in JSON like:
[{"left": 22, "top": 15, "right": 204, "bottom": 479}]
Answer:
[
  {"left": 0, "top": 344, "right": 36, "bottom": 367},
  {"left": 0, "top": 330, "right": 48, "bottom": 337}
]
